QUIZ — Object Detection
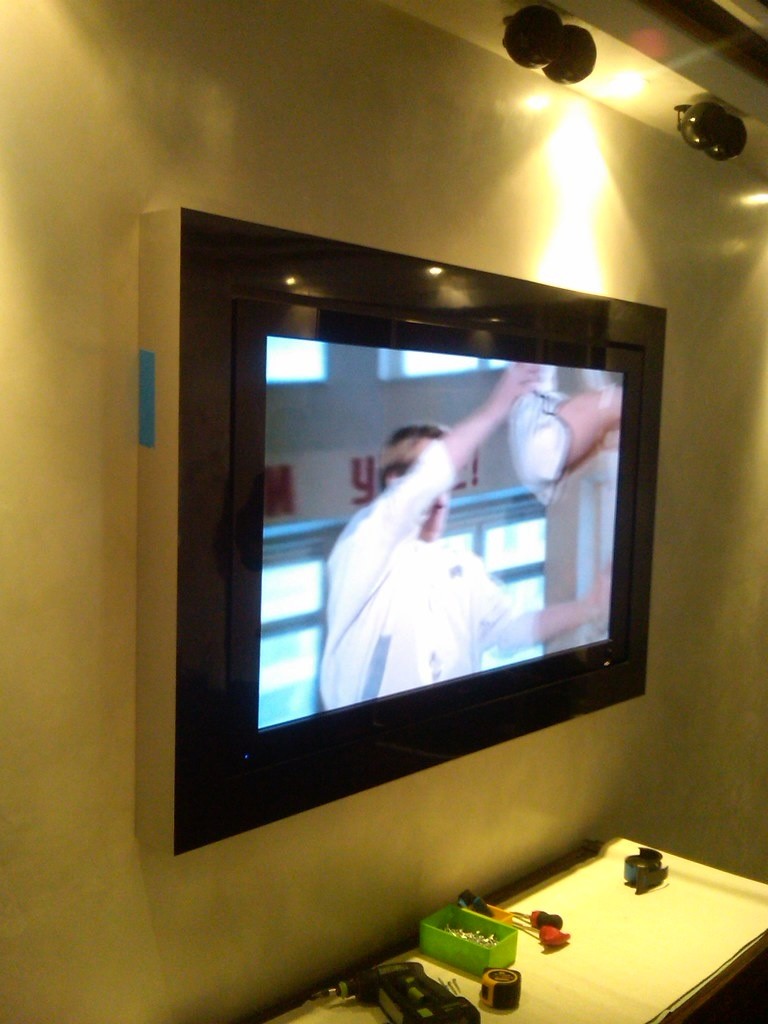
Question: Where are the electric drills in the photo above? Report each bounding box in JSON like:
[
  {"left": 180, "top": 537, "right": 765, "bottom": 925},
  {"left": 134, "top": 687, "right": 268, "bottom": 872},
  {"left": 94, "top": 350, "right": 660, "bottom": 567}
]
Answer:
[{"left": 308, "top": 960, "right": 480, "bottom": 1024}]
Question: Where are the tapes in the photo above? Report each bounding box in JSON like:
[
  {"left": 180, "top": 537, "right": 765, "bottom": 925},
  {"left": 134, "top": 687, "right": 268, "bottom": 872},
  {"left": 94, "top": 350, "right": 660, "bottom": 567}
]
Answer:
[{"left": 457, "top": 890, "right": 476, "bottom": 906}]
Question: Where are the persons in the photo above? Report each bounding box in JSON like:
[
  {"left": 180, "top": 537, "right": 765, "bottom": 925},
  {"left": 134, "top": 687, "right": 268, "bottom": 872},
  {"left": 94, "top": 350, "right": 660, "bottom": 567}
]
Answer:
[{"left": 319, "top": 360, "right": 623, "bottom": 710}]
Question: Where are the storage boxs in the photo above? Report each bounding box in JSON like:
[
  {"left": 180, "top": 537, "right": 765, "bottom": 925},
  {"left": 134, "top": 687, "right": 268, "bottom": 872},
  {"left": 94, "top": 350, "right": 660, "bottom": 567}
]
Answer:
[{"left": 418, "top": 904, "right": 518, "bottom": 975}]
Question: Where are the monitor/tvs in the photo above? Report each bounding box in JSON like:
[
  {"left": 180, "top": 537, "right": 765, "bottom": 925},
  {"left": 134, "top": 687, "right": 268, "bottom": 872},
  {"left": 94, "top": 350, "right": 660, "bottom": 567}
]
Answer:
[{"left": 132, "top": 209, "right": 668, "bottom": 860}]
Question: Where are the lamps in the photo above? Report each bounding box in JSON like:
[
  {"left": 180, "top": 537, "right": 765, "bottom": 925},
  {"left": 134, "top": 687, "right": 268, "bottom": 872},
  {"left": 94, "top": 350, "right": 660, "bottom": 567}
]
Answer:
[
  {"left": 673, "top": 103, "right": 747, "bottom": 162},
  {"left": 498, "top": 2, "right": 597, "bottom": 87}
]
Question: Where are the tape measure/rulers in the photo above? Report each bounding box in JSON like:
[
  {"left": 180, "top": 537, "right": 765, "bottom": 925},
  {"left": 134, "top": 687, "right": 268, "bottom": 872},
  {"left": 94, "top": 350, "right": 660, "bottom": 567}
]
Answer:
[{"left": 479, "top": 966, "right": 521, "bottom": 1009}]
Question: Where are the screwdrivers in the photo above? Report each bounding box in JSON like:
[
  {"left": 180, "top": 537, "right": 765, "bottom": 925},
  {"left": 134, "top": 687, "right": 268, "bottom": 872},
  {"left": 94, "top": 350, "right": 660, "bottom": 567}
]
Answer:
[{"left": 504, "top": 908, "right": 563, "bottom": 929}]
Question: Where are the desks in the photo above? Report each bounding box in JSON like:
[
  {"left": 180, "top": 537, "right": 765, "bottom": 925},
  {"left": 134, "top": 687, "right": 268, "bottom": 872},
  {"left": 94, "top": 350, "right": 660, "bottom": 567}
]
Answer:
[{"left": 239, "top": 835, "right": 768, "bottom": 1024}]
